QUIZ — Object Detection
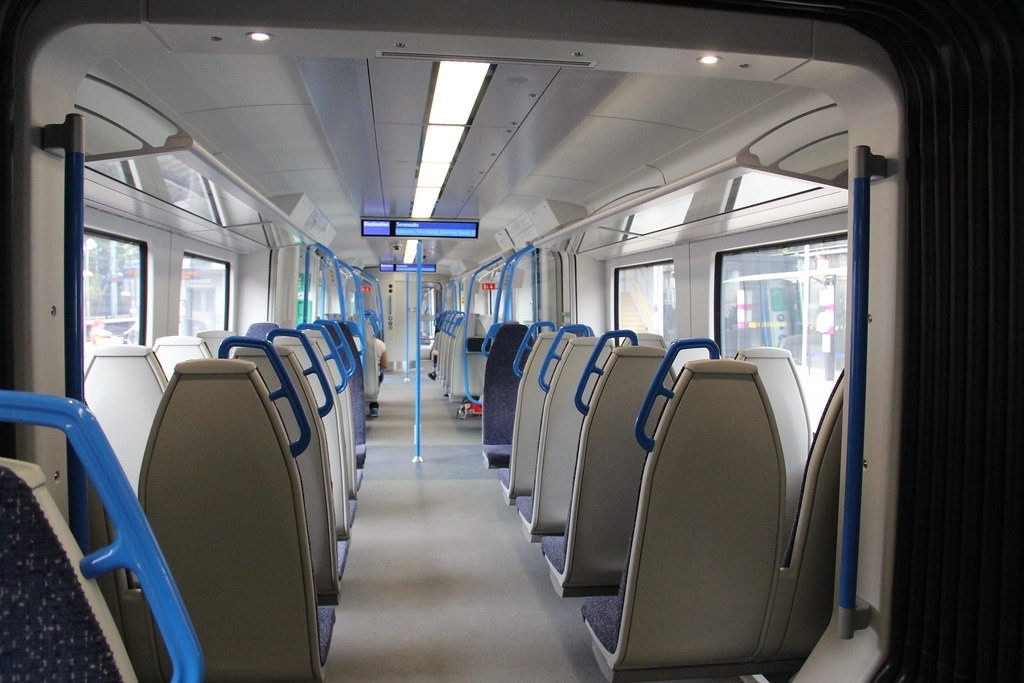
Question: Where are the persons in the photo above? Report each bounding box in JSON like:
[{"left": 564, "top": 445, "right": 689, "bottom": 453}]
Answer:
[
  {"left": 369, "top": 339, "right": 388, "bottom": 416},
  {"left": 428, "top": 331, "right": 441, "bottom": 380}
]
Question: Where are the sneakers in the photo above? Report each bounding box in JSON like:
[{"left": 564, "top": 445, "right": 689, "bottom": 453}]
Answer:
[{"left": 370, "top": 407, "right": 378, "bottom": 415}]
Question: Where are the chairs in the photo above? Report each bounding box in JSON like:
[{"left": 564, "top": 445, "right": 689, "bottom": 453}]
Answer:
[
  {"left": 150, "top": 335, "right": 213, "bottom": 380},
  {"left": 433, "top": 310, "right": 466, "bottom": 394},
  {"left": 324, "top": 321, "right": 367, "bottom": 469},
  {"left": 313, "top": 318, "right": 365, "bottom": 501},
  {"left": 83, "top": 345, "right": 168, "bottom": 496},
  {"left": 481, "top": 323, "right": 536, "bottom": 469},
  {"left": 539, "top": 327, "right": 678, "bottom": 600},
  {"left": 229, "top": 329, "right": 350, "bottom": 606},
  {"left": 581, "top": 338, "right": 787, "bottom": 683},
  {"left": 762, "top": 367, "right": 844, "bottom": 683},
  {"left": 447, "top": 315, "right": 492, "bottom": 403},
  {"left": 346, "top": 313, "right": 381, "bottom": 403},
  {"left": 83, "top": 400, "right": 151, "bottom": 683},
  {"left": 0, "top": 390, "right": 207, "bottom": 683},
  {"left": 136, "top": 335, "right": 336, "bottom": 683},
  {"left": 733, "top": 347, "right": 815, "bottom": 560},
  {"left": 265, "top": 323, "right": 358, "bottom": 540},
  {"left": 515, "top": 324, "right": 614, "bottom": 545},
  {"left": 245, "top": 324, "right": 279, "bottom": 339},
  {"left": 496, "top": 321, "right": 579, "bottom": 507}
]
